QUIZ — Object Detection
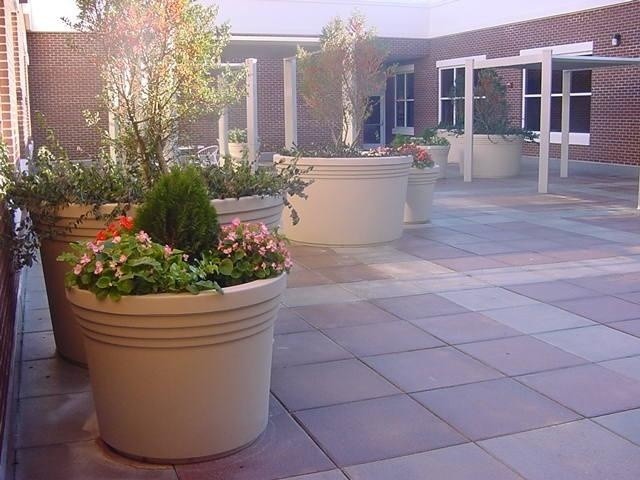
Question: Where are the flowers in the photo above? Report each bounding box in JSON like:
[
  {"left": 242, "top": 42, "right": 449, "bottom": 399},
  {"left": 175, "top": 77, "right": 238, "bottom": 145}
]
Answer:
[
  {"left": 54, "top": 163, "right": 294, "bottom": 302},
  {"left": 368, "top": 142, "right": 434, "bottom": 170}
]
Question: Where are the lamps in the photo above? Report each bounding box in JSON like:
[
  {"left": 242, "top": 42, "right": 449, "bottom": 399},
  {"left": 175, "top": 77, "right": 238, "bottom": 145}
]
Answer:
[{"left": 612, "top": 34, "right": 621, "bottom": 46}]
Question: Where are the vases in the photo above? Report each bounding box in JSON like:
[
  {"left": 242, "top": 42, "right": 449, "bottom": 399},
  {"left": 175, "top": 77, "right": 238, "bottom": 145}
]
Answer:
[
  {"left": 64, "top": 264, "right": 286, "bottom": 464},
  {"left": 404, "top": 165, "right": 440, "bottom": 224}
]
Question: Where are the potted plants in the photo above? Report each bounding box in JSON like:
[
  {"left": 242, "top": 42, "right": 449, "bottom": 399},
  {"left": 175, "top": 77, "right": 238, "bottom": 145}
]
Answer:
[
  {"left": 218, "top": 126, "right": 260, "bottom": 175},
  {"left": 414, "top": 127, "right": 450, "bottom": 180},
  {"left": 0, "top": 1, "right": 316, "bottom": 369},
  {"left": 272, "top": 8, "right": 414, "bottom": 247},
  {"left": 458, "top": 68, "right": 540, "bottom": 177},
  {"left": 435, "top": 70, "right": 465, "bottom": 164}
]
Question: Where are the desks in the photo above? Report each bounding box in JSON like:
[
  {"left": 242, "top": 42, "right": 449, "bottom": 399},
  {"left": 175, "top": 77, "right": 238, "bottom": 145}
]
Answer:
[{"left": 176, "top": 144, "right": 205, "bottom": 158}]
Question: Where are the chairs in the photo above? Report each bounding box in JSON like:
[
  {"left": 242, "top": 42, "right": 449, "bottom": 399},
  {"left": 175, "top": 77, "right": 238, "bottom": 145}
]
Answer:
[{"left": 193, "top": 144, "right": 218, "bottom": 164}]
